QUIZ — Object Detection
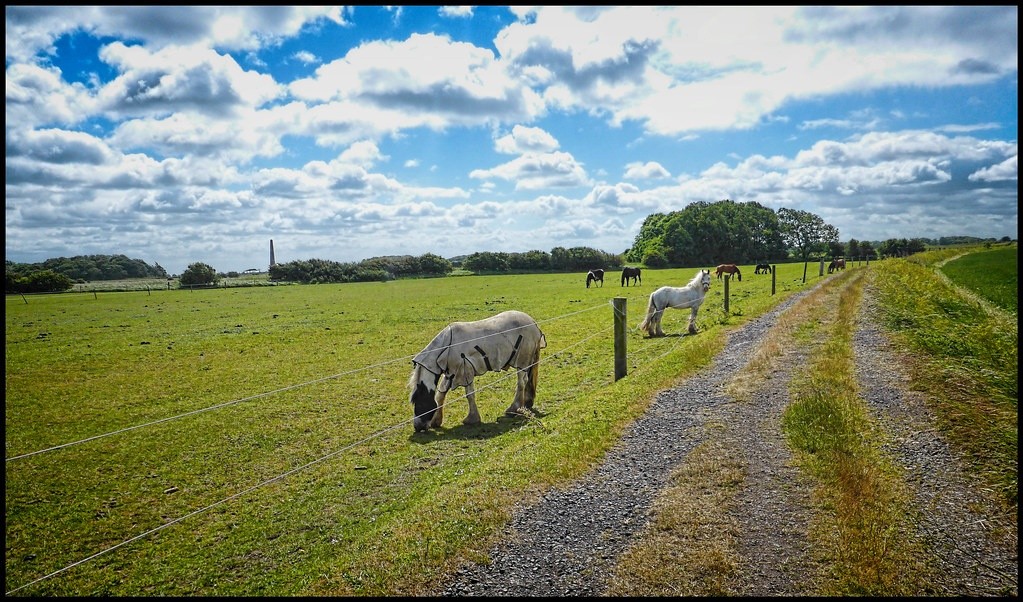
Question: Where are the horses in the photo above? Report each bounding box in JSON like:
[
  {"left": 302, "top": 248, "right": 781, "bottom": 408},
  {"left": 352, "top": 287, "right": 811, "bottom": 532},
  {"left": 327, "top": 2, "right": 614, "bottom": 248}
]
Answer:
[
  {"left": 754, "top": 264, "right": 771, "bottom": 274},
  {"left": 620, "top": 267, "right": 641, "bottom": 287},
  {"left": 586, "top": 269, "right": 604, "bottom": 288},
  {"left": 408, "top": 310, "right": 541, "bottom": 433},
  {"left": 641, "top": 269, "right": 710, "bottom": 336},
  {"left": 712, "top": 264, "right": 741, "bottom": 281},
  {"left": 827, "top": 259, "right": 846, "bottom": 273}
]
